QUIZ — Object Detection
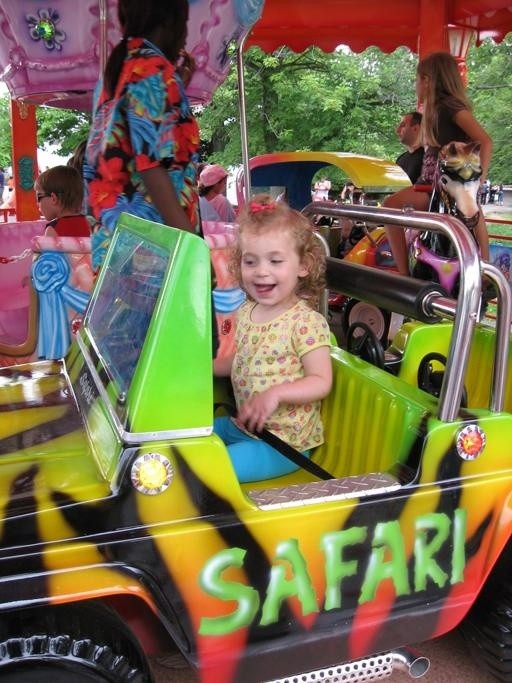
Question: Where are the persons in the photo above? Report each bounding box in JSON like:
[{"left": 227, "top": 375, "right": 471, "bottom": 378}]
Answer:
[
  {"left": 82, "top": 1, "right": 198, "bottom": 284},
  {"left": 0, "top": 166, "right": 6, "bottom": 205},
  {"left": 383, "top": 50, "right": 490, "bottom": 270},
  {"left": 314, "top": 175, "right": 332, "bottom": 201},
  {"left": 7, "top": 176, "right": 14, "bottom": 193},
  {"left": 341, "top": 181, "right": 364, "bottom": 204},
  {"left": 214, "top": 193, "right": 333, "bottom": 485},
  {"left": 396, "top": 112, "right": 424, "bottom": 184},
  {"left": 480, "top": 179, "right": 504, "bottom": 206},
  {"left": 198, "top": 164, "right": 235, "bottom": 223},
  {"left": 33, "top": 165, "right": 89, "bottom": 237}
]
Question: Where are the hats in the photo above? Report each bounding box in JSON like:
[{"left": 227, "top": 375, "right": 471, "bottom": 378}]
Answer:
[{"left": 199, "top": 165, "right": 228, "bottom": 187}]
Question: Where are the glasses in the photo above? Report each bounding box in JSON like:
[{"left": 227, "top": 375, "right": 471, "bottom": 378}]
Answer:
[{"left": 36, "top": 194, "right": 53, "bottom": 202}]
